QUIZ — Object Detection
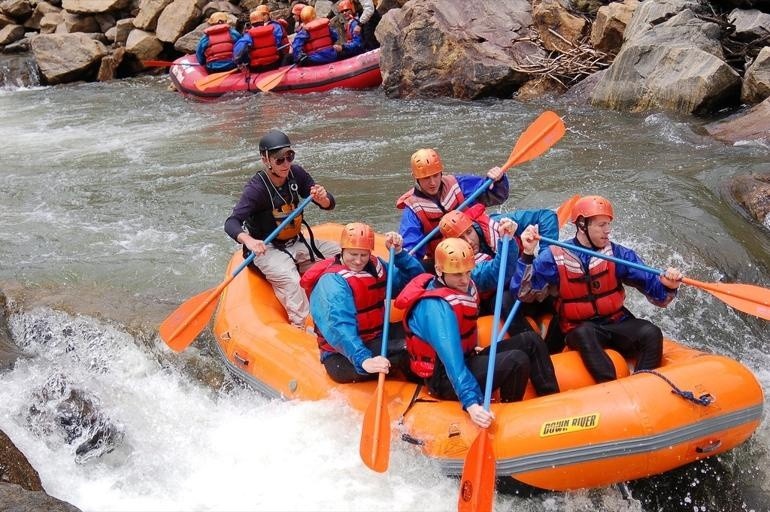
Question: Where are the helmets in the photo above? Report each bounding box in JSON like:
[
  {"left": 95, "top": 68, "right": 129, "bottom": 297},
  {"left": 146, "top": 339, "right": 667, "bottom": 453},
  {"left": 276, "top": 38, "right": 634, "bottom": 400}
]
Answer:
[
  {"left": 410, "top": 147, "right": 444, "bottom": 179},
  {"left": 339, "top": 221, "right": 375, "bottom": 251},
  {"left": 570, "top": 194, "right": 615, "bottom": 223},
  {"left": 207, "top": 11, "right": 228, "bottom": 25},
  {"left": 438, "top": 209, "right": 474, "bottom": 239},
  {"left": 434, "top": 236, "right": 476, "bottom": 274},
  {"left": 257, "top": 129, "right": 292, "bottom": 152},
  {"left": 249, "top": 4, "right": 270, "bottom": 26},
  {"left": 337, "top": 0, "right": 353, "bottom": 14},
  {"left": 291, "top": 3, "right": 317, "bottom": 23}
]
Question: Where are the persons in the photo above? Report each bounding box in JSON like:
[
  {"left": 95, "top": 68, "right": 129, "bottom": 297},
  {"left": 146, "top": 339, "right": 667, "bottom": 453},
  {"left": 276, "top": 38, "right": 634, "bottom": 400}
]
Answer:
[
  {"left": 299, "top": 147, "right": 567, "bottom": 431},
  {"left": 226, "top": 130, "right": 342, "bottom": 329},
  {"left": 196, "top": 1, "right": 381, "bottom": 75},
  {"left": 508, "top": 196, "right": 684, "bottom": 383}
]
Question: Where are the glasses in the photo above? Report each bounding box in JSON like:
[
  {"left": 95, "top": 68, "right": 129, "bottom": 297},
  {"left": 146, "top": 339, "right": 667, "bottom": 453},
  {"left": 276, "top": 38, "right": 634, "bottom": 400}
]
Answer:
[{"left": 268, "top": 149, "right": 295, "bottom": 166}]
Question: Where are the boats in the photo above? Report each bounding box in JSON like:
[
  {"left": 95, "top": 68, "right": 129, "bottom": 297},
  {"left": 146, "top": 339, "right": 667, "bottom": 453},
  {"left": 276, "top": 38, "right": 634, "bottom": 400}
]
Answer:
[
  {"left": 169, "top": 38, "right": 383, "bottom": 102},
  {"left": 212, "top": 221, "right": 764, "bottom": 500}
]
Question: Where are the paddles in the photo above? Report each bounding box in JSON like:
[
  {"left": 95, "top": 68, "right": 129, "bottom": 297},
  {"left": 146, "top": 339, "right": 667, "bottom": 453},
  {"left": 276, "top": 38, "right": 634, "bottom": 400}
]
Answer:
[
  {"left": 158, "top": 195, "right": 314, "bottom": 352},
  {"left": 256, "top": 57, "right": 308, "bottom": 92},
  {"left": 142, "top": 61, "right": 201, "bottom": 67},
  {"left": 193, "top": 42, "right": 292, "bottom": 90},
  {"left": 533, "top": 232, "right": 769, "bottom": 322},
  {"left": 457, "top": 230, "right": 510, "bottom": 511},
  {"left": 495, "top": 194, "right": 580, "bottom": 341},
  {"left": 407, "top": 111, "right": 565, "bottom": 256},
  {"left": 359, "top": 245, "right": 395, "bottom": 474}
]
